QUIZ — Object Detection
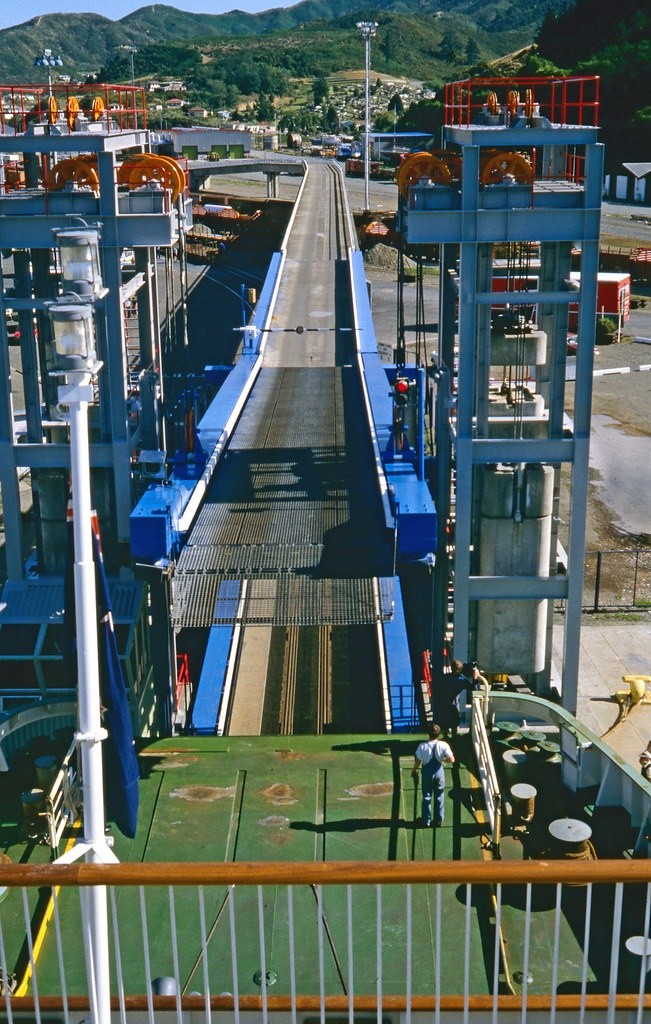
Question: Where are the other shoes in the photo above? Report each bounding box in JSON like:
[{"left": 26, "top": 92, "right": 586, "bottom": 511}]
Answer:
[
  {"left": 418, "top": 817, "right": 430, "bottom": 827},
  {"left": 431, "top": 822, "right": 441, "bottom": 827}
]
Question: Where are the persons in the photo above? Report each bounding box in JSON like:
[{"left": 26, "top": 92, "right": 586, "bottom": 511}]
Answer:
[
  {"left": 639, "top": 740, "right": 651, "bottom": 783},
  {"left": 434, "top": 660, "right": 480, "bottom": 741},
  {"left": 413, "top": 725, "right": 456, "bottom": 827}
]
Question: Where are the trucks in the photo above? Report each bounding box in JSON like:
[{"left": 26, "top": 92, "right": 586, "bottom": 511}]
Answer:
[{"left": 446, "top": 256, "right": 631, "bottom": 337}]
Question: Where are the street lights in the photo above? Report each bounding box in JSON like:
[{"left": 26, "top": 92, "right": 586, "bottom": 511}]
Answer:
[
  {"left": 127, "top": 45, "right": 138, "bottom": 130},
  {"left": 354, "top": 21, "right": 381, "bottom": 213},
  {"left": 31, "top": 47, "right": 64, "bottom": 97}
]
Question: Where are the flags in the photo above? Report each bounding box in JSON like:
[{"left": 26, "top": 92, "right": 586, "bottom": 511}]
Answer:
[{"left": 65, "top": 481, "right": 140, "bottom": 838}]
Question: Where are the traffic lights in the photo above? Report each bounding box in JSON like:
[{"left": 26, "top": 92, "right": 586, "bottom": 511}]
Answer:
[{"left": 394, "top": 375, "right": 410, "bottom": 406}]
